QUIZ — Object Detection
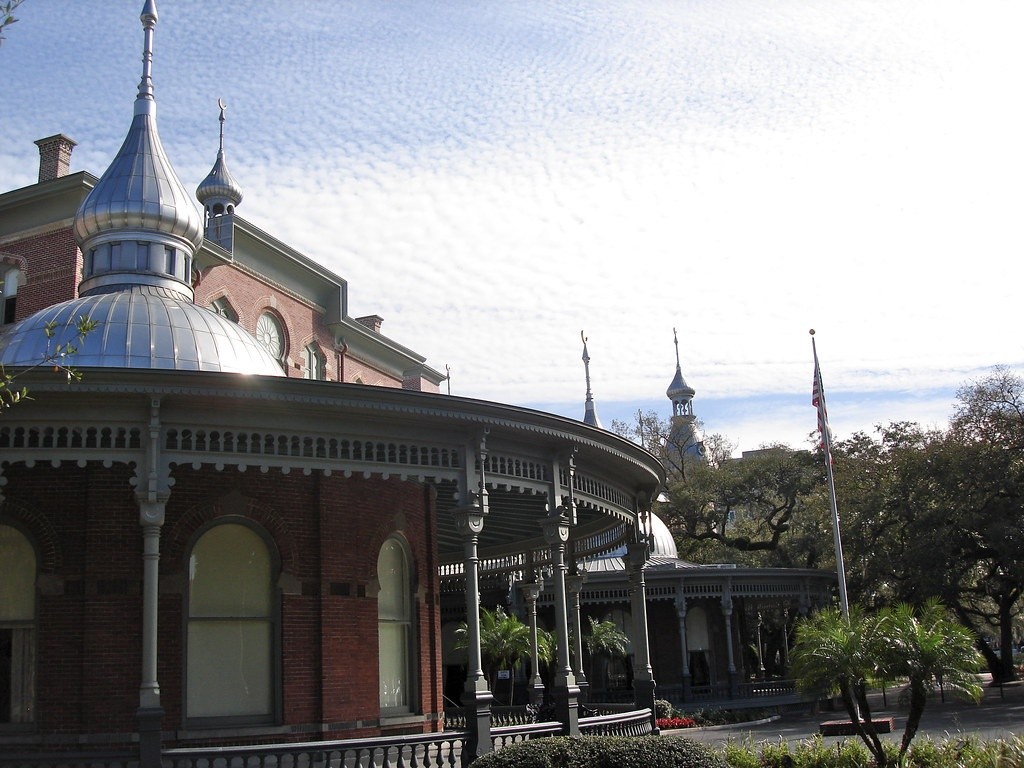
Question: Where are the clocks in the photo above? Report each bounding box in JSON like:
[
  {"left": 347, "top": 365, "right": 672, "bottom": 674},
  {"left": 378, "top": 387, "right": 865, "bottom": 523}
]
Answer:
[{"left": 699, "top": 445, "right": 706, "bottom": 456}]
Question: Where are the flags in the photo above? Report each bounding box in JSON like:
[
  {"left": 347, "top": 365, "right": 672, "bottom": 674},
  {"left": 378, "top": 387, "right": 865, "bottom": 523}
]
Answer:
[{"left": 811, "top": 356, "right": 833, "bottom": 466}]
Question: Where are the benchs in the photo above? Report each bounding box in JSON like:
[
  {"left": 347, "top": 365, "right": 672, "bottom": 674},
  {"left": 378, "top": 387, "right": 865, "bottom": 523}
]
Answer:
[{"left": 526, "top": 703, "right": 601, "bottom": 739}]
